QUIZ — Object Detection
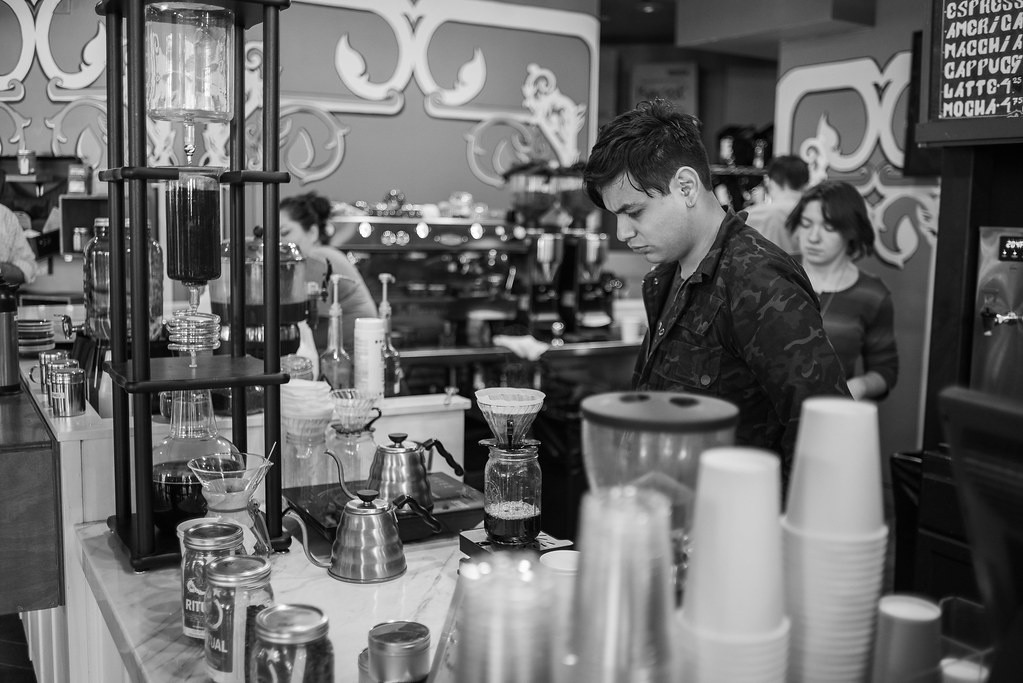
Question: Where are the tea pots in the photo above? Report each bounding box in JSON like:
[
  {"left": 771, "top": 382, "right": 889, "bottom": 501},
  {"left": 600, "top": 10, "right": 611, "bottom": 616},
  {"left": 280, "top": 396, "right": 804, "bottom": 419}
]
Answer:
[
  {"left": 282, "top": 489, "right": 447, "bottom": 585},
  {"left": 324, "top": 431, "right": 466, "bottom": 512}
]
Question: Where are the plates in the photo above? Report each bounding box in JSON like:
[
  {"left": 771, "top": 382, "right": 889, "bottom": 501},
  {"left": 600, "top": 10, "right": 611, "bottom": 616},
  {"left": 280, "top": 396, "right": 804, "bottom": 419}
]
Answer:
[{"left": 15, "top": 319, "right": 56, "bottom": 356}]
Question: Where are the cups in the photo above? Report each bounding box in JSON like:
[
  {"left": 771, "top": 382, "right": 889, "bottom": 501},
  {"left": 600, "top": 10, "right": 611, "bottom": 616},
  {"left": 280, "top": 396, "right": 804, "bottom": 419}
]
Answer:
[{"left": 440, "top": 396, "right": 945, "bottom": 683}]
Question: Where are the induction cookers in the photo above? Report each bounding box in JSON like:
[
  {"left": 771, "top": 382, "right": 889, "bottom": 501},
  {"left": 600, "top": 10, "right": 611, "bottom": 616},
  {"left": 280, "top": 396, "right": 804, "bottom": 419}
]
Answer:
[{"left": 280, "top": 472, "right": 486, "bottom": 544}]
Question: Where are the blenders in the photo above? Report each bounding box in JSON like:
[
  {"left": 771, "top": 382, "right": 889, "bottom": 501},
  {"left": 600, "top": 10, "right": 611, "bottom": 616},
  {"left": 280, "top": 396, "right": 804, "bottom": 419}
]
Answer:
[{"left": 505, "top": 164, "right": 622, "bottom": 341}]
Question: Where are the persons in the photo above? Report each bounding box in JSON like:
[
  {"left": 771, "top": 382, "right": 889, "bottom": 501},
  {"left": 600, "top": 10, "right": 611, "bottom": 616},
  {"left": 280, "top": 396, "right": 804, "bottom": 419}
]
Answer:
[
  {"left": 583, "top": 97, "right": 901, "bottom": 509},
  {"left": 278, "top": 192, "right": 377, "bottom": 356},
  {"left": 0, "top": 203, "right": 38, "bottom": 289}
]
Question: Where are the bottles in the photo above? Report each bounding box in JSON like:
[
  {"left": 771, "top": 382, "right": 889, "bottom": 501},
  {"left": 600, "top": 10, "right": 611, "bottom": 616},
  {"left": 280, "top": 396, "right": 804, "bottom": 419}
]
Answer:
[
  {"left": 29, "top": 351, "right": 86, "bottom": 417},
  {"left": 484, "top": 448, "right": 545, "bottom": 546},
  {"left": 80, "top": 216, "right": 163, "bottom": 342},
  {"left": 327, "top": 419, "right": 378, "bottom": 503},
  {"left": 151, "top": 390, "right": 246, "bottom": 531},
  {"left": 354, "top": 319, "right": 388, "bottom": 405},
  {"left": 281, "top": 431, "right": 327, "bottom": 495},
  {"left": 188, "top": 450, "right": 271, "bottom": 554}
]
